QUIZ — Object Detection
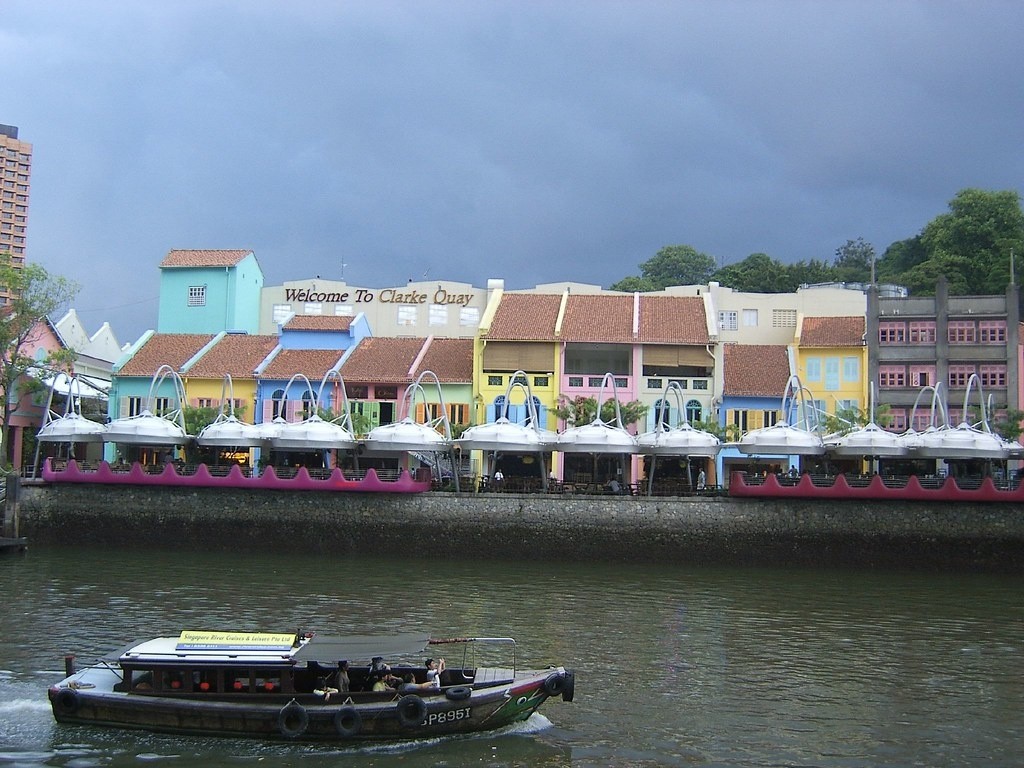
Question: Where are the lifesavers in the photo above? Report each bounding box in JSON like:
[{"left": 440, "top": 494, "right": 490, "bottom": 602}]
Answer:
[
  {"left": 52, "top": 687, "right": 83, "bottom": 717},
  {"left": 445, "top": 686, "right": 472, "bottom": 701},
  {"left": 544, "top": 672, "right": 564, "bottom": 697},
  {"left": 333, "top": 706, "right": 363, "bottom": 737},
  {"left": 562, "top": 669, "right": 575, "bottom": 702},
  {"left": 278, "top": 705, "right": 309, "bottom": 739},
  {"left": 395, "top": 693, "right": 428, "bottom": 730}
]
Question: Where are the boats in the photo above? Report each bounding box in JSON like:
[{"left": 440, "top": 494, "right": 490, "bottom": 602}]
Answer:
[{"left": 47, "top": 629, "right": 578, "bottom": 743}]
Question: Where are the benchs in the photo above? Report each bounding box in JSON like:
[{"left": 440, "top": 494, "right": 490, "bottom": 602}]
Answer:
[
  {"left": 51, "top": 456, "right": 400, "bottom": 482},
  {"left": 743, "top": 473, "right": 1023, "bottom": 491},
  {"left": 437, "top": 474, "right": 721, "bottom": 497}
]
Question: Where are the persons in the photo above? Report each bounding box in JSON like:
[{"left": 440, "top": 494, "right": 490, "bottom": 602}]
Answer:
[
  {"left": 607, "top": 478, "right": 620, "bottom": 492},
  {"left": 697, "top": 468, "right": 705, "bottom": 489},
  {"left": 399, "top": 467, "right": 403, "bottom": 478},
  {"left": 177, "top": 458, "right": 185, "bottom": 470},
  {"left": 334, "top": 661, "right": 350, "bottom": 692},
  {"left": 368, "top": 657, "right": 390, "bottom": 690},
  {"left": 425, "top": 658, "right": 445, "bottom": 688},
  {"left": 386, "top": 670, "right": 403, "bottom": 687},
  {"left": 494, "top": 469, "right": 503, "bottom": 486},
  {"left": 548, "top": 468, "right": 555, "bottom": 480},
  {"left": 412, "top": 467, "right": 415, "bottom": 479},
  {"left": 164, "top": 452, "right": 173, "bottom": 466},
  {"left": 398, "top": 673, "right": 431, "bottom": 689},
  {"left": 373, "top": 671, "right": 396, "bottom": 692},
  {"left": 789, "top": 465, "right": 798, "bottom": 486},
  {"left": 777, "top": 469, "right": 785, "bottom": 476}
]
